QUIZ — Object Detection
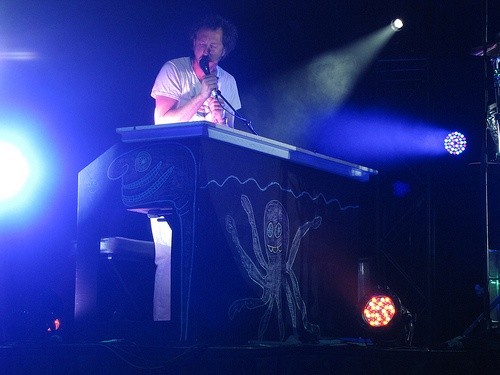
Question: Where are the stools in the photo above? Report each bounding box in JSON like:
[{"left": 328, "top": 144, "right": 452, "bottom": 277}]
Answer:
[{"left": 68, "top": 237, "right": 156, "bottom": 337}]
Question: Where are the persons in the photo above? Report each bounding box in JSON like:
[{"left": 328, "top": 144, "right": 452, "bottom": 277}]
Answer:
[{"left": 150, "top": 16, "right": 243, "bottom": 321}]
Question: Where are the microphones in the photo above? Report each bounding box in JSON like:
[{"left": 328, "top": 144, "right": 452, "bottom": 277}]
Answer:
[{"left": 200, "top": 58, "right": 217, "bottom": 98}]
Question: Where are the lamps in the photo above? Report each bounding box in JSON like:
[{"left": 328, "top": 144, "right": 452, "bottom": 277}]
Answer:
[
  {"left": 443, "top": 131, "right": 468, "bottom": 156},
  {"left": 359, "top": 289, "right": 415, "bottom": 350},
  {"left": 390, "top": 18, "right": 404, "bottom": 31}
]
